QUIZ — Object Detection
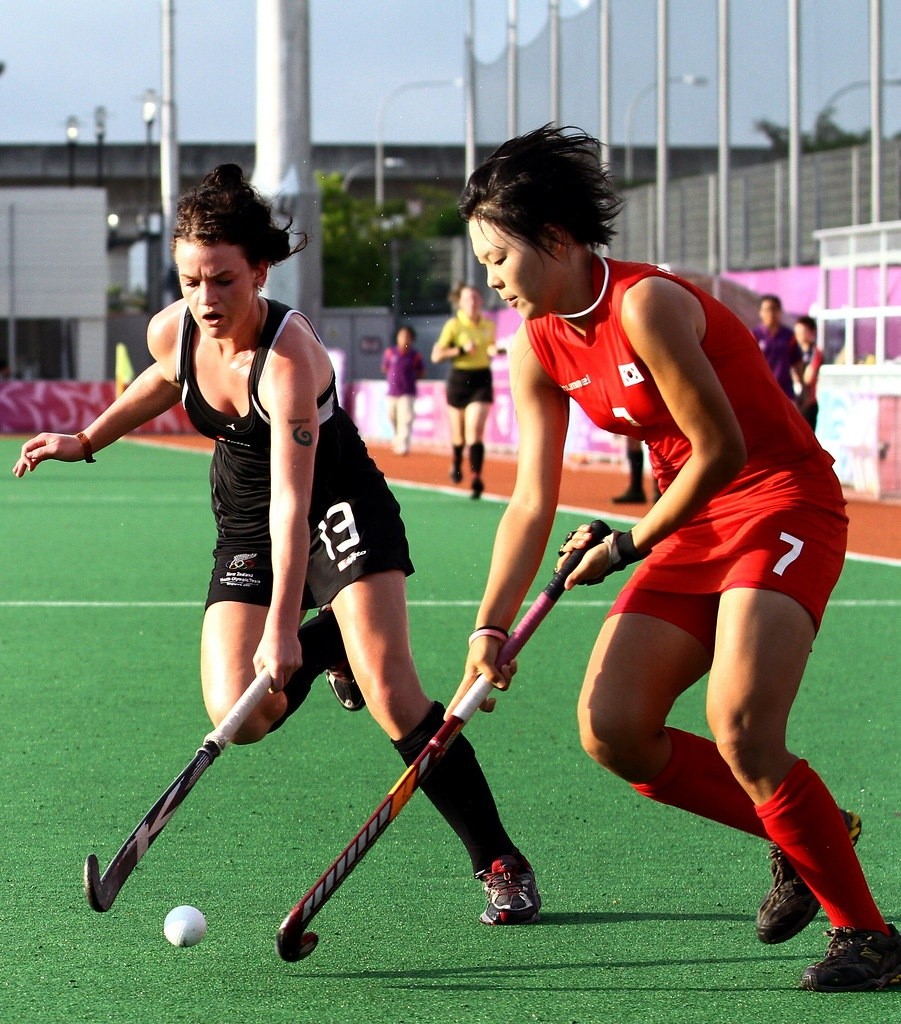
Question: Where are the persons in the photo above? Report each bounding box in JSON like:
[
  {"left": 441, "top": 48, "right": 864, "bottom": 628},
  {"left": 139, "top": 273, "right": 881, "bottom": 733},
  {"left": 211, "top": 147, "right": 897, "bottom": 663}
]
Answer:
[
  {"left": 443, "top": 121, "right": 901, "bottom": 995},
  {"left": 430, "top": 282, "right": 507, "bottom": 499},
  {"left": 612, "top": 435, "right": 660, "bottom": 505},
  {"left": 381, "top": 325, "right": 424, "bottom": 457},
  {"left": 13, "top": 165, "right": 541, "bottom": 927},
  {"left": 749, "top": 295, "right": 804, "bottom": 411},
  {"left": 789, "top": 316, "right": 822, "bottom": 434}
]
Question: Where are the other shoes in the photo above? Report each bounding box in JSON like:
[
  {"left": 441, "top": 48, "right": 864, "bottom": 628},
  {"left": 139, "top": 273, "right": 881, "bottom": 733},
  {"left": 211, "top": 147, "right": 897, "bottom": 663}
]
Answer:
[
  {"left": 452, "top": 462, "right": 463, "bottom": 481},
  {"left": 614, "top": 488, "right": 646, "bottom": 504},
  {"left": 469, "top": 479, "right": 485, "bottom": 499}
]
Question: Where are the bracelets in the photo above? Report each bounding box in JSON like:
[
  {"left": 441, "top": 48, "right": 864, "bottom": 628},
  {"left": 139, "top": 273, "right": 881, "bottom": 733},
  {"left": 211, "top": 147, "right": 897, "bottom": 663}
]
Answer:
[
  {"left": 469, "top": 625, "right": 508, "bottom": 646},
  {"left": 75, "top": 432, "right": 96, "bottom": 463},
  {"left": 458, "top": 345, "right": 464, "bottom": 355}
]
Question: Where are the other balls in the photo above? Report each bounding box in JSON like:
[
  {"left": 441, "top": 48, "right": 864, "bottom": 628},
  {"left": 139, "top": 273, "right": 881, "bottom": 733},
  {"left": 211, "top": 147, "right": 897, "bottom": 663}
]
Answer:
[{"left": 163, "top": 905, "right": 206, "bottom": 946}]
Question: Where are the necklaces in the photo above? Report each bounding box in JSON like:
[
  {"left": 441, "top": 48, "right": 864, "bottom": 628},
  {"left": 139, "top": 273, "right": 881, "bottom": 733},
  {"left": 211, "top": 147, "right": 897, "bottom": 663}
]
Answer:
[{"left": 550, "top": 252, "right": 609, "bottom": 319}]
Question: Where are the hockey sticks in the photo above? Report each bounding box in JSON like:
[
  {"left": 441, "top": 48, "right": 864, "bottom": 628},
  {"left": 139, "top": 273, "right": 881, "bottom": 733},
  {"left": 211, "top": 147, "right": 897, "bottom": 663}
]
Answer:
[
  {"left": 80, "top": 669, "right": 272, "bottom": 912},
  {"left": 272, "top": 520, "right": 613, "bottom": 962}
]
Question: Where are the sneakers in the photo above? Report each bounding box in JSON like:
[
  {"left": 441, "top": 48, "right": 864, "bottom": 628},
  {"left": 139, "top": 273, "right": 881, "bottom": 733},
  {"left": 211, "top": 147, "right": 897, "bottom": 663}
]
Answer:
[
  {"left": 755, "top": 806, "right": 861, "bottom": 943},
  {"left": 476, "top": 855, "right": 541, "bottom": 924},
  {"left": 802, "top": 924, "right": 901, "bottom": 990},
  {"left": 318, "top": 604, "right": 366, "bottom": 711}
]
